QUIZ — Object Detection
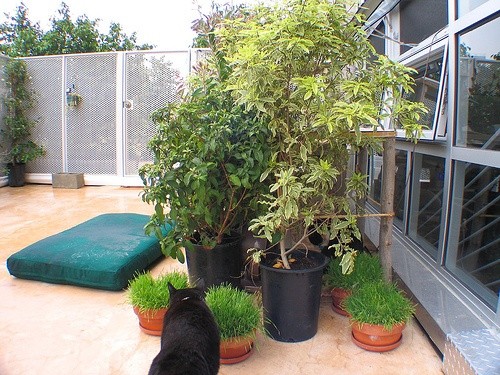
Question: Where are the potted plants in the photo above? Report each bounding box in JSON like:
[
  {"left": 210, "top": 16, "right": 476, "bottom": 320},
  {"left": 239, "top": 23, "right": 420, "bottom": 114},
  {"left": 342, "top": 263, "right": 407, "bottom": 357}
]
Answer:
[
  {"left": 0, "top": 56, "right": 47, "bottom": 187},
  {"left": 323, "top": 251, "right": 385, "bottom": 317},
  {"left": 123, "top": 268, "right": 201, "bottom": 336},
  {"left": 339, "top": 277, "right": 419, "bottom": 352},
  {"left": 206, "top": 0, "right": 431, "bottom": 343},
  {"left": 139, "top": 30, "right": 277, "bottom": 292},
  {"left": 204, "top": 280, "right": 279, "bottom": 364}
]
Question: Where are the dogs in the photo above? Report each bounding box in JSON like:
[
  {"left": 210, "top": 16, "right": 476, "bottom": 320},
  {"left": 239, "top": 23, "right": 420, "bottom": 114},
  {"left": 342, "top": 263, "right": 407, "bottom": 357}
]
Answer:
[
  {"left": 146, "top": 280, "right": 222, "bottom": 375},
  {"left": 306, "top": 221, "right": 365, "bottom": 275}
]
